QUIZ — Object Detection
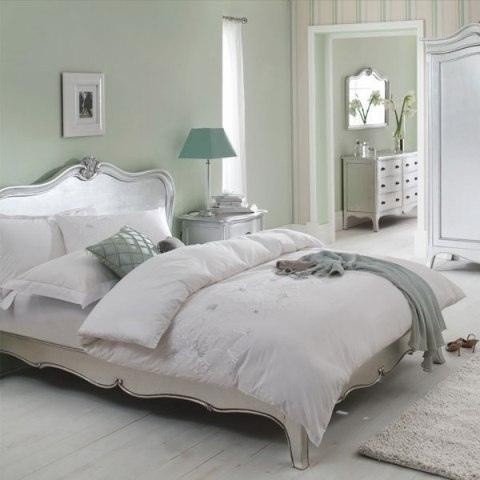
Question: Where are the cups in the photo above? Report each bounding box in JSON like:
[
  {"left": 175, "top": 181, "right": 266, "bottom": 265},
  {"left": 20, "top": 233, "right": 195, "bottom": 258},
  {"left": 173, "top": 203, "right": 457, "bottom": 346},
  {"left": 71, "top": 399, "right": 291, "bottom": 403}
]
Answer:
[{"left": 367, "top": 146, "right": 376, "bottom": 159}]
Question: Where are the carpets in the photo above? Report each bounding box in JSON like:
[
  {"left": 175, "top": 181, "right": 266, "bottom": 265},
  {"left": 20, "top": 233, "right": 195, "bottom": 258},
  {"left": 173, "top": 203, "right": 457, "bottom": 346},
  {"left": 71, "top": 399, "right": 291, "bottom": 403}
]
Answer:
[{"left": 356, "top": 351, "right": 480, "bottom": 479}]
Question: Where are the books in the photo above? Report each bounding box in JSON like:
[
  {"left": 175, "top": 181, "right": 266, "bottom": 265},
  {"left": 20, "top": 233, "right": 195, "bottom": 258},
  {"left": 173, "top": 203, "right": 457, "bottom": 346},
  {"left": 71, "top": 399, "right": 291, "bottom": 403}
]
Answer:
[{"left": 208, "top": 193, "right": 257, "bottom": 214}]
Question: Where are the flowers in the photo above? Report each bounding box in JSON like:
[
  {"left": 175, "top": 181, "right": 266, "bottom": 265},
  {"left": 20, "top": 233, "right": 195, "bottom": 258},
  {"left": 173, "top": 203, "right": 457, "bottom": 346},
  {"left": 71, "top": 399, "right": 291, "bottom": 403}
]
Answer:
[
  {"left": 349, "top": 89, "right": 384, "bottom": 125},
  {"left": 385, "top": 93, "right": 414, "bottom": 137}
]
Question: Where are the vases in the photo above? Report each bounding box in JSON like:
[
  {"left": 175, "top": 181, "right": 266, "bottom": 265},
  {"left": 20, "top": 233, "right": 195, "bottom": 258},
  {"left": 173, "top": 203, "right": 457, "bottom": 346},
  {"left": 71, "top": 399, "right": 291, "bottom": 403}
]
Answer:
[{"left": 394, "top": 140, "right": 408, "bottom": 154}]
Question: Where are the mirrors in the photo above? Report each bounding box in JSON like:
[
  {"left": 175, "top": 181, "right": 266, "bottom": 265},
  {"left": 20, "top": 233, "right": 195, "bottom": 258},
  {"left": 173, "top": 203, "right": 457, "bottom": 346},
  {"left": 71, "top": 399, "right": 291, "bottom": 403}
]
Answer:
[{"left": 344, "top": 68, "right": 390, "bottom": 131}]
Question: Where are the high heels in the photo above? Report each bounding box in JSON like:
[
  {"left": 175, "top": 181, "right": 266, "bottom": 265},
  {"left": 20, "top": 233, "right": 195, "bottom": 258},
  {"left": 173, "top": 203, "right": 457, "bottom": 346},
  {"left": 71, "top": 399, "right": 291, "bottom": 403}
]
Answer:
[
  {"left": 455, "top": 334, "right": 479, "bottom": 353},
  {"left": 446, "top": 340, "right": 462, "bottom": 357}
]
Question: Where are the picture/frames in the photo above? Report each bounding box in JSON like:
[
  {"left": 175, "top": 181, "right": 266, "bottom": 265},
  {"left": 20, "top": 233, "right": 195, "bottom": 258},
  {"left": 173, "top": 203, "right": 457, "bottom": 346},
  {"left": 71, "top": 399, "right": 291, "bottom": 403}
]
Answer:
[{"left": 61, "top": 72, "right": 104, "bottom": 139}]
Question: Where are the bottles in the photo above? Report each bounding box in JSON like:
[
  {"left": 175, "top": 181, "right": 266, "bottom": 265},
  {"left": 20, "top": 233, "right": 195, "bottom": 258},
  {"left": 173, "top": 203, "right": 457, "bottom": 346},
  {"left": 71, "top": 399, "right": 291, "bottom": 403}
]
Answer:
[
  {"left": 355, "top": 141, "right": 361, "bottom": 160},
  {"left": 362, "top": 142, "right": 368, "bottom": 158}
]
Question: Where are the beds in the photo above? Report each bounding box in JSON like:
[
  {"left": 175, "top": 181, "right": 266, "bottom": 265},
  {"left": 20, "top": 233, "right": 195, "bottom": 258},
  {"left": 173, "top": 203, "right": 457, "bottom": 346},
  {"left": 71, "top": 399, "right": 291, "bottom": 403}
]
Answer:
[{"left": 0, "top": 156, "right": 466, "bottom": 469}]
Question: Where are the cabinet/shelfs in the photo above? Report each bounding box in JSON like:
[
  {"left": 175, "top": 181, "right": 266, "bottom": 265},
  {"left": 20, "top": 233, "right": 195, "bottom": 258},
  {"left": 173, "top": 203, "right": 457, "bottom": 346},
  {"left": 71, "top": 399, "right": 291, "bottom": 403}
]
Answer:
[
  {"left": 420, "top": 21, "right": 479, "bottom": 271},
  {"left": 403, "top": 152, "right": 418, "bottom": 214},
  {"left": 340, "top": 156, "right": 403, "bottom": 232}
]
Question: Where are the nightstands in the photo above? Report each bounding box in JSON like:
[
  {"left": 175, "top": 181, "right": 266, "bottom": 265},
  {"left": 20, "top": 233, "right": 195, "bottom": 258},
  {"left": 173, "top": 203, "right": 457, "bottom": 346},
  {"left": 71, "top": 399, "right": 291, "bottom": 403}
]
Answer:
[{"left": 176, "top": 208, "right": 268, "bottom": 248}]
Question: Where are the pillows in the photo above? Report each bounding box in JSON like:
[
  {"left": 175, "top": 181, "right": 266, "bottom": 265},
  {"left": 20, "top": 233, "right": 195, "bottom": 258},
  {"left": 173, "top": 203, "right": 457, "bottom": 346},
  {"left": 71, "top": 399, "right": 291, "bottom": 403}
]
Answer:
[
  {"left": 86, "top": 225, "right": 157, "bottom": 280},
  {"left": 155, "top": 236, "right": 187, "bottom": 253},
  {"left": 1, "top": 208, "right": 88, "bottom": 282},
  {"left": 55, "top": 207, "right": 176, "bottom": 260},
  {"left": 1, "top": 246, "right": 119, "bottom": 310}
]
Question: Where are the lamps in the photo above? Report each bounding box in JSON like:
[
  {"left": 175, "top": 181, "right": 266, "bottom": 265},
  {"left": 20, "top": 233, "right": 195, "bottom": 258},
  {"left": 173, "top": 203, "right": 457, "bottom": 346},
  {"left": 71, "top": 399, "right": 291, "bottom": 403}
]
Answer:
[{"left": 178, "top": 127, "right": 236, "bottom": 218}]
